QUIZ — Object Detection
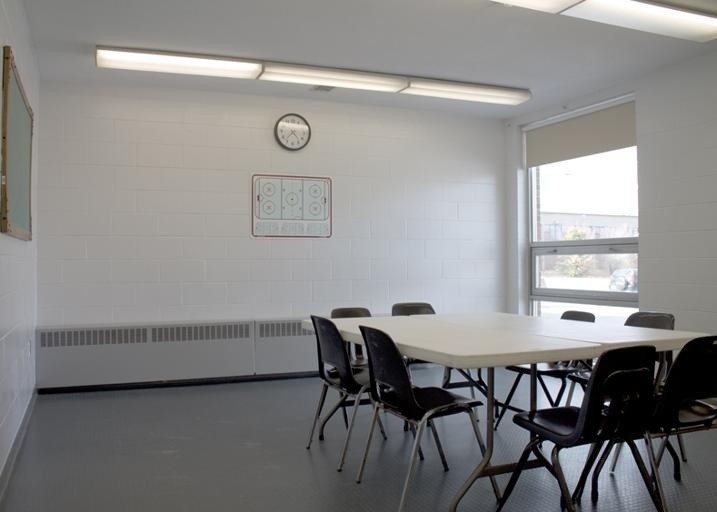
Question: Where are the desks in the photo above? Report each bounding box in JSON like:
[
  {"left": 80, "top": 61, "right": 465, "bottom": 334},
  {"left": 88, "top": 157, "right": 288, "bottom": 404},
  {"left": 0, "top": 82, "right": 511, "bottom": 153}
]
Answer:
[{"left": 303, "top": 310, "right": 713, "bottom": 509}]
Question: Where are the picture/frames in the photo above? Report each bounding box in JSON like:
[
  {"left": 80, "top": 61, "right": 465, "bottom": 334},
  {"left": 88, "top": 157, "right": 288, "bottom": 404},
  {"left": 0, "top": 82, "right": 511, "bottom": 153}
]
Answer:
[{"left": 1, "top": 45, "right": 35, "bottom": 242}]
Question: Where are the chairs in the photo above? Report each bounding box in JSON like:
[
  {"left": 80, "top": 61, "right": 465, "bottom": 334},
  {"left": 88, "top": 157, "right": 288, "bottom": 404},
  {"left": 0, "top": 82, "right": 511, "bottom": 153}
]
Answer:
[
  {"left": 564, "top": 311, "right": 690, "bottom": 475},
  {"left": 493, "top": 310, "right": 597, "bottom": 431},
  {"left": 357, "top": 323, "right": 500, "bottom": 511},
  {"left": 331, "top": 307, "right": 399, "bottom": 428},
  {"left": 306, "top": 313, "right": 426, "bottom": 471},
  {"left": 393, "top": 304, "right": 476, "bottom": 425},
  {"left": 497, "top": 345, "right": 654, "bottom": 512},
  {"left": 595, "top": 337, "right": 717, "bottom": 512}
]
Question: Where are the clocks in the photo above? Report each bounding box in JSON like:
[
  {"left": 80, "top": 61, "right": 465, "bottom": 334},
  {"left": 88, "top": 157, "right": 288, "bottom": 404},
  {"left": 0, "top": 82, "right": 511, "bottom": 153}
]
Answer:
[{"left": 273, "top": 113, "right": 311, "bottom": 151}]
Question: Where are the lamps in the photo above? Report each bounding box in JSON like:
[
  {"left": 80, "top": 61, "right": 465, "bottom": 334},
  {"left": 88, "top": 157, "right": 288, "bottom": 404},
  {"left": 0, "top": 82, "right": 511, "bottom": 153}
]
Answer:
[
  {"left": 497, "top": 0, "right": 714, "bottom": 50},
  {"left": 91, "top": 45, "right": 533, "bottom": 108}
]
